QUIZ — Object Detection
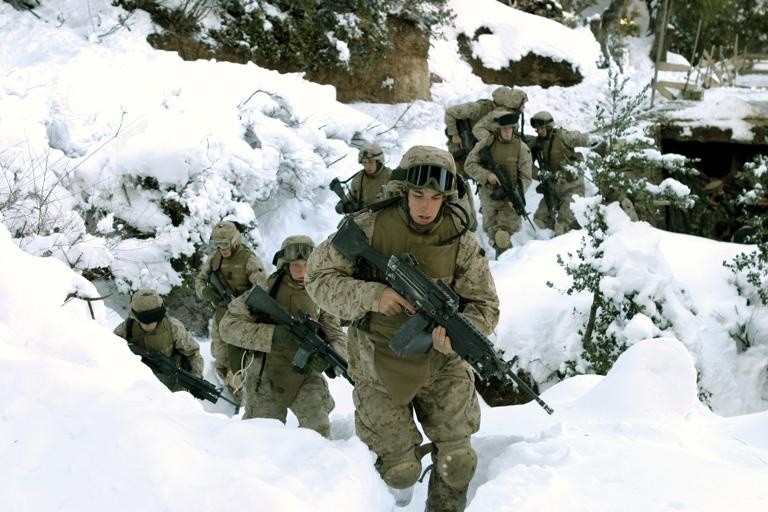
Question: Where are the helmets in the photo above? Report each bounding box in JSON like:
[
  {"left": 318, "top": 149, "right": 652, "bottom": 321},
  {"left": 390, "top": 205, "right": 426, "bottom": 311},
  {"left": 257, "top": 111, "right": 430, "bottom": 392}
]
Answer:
[
  {"left": 531, "top": 111, "right": 556, "bottom": 128},
  {"left": 129, "top": 290, "right": 166, "bottom": 325},
  {"left": 277, "top": 235, "right": 314, "bottom": 268},
  {"left": 359, "top": 144, "right": 385, "bottom": 165},
  {"left": 209, "top": 221, "right": 243, "bottom": 253},
  {"left": 387, "top": 145, "right": 459, "bottom": 204},
  {"left": 491, "top": 88, "right": 528, "bottom": 128}
]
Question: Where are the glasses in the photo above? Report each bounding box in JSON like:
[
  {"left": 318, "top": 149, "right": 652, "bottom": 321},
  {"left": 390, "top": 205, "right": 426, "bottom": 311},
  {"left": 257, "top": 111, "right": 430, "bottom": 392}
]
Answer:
[
  {"left": 406, "top": 164, "right": 454, "bottom": 193},
  {"left": 283, "top": 243, "right": 313, "bottom": 261},
  {"left": 138, "top": 308, "right": 164, "bottom": 323},
  {"left": 210, "top": 239, "right": 231, "bottom": 250}
]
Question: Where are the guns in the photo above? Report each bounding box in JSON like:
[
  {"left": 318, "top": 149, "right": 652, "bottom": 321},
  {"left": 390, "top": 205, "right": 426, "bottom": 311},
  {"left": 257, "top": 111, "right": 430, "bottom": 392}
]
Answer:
[
  {"left": 531, "top": 148, "right": 559, "bottom": 220},
  {"left": 331, "top": 177, "right": 359, "bottom": 213},
  {"left": 478, "top": 146, "right": 537, "bottom": 234},
  {"left": 129, "top": 344, "right": 241, "bottom": 410},
  {"left": 246, "top": 286, "right": 355, "bottom": 388},
  {"left": 447, "top": 120, "right": 476, "bottom": 160},
  {"left": 330, "top": 219, "right": 553, "bottom": 415},
  {"left": 209, "top": 273, "right": 235, "bottom": 311}
]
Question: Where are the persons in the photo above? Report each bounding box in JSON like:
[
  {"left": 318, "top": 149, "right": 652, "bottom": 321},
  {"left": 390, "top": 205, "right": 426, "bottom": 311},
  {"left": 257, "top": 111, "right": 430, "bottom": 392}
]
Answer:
[
  {"left": 464, "top": 110, "right": 533, "bottom": 263},
  {"left": 328, "top": 148, "right": 400, "bottom": 214},
  {"left": 221, "top": 235, "right": 352, "bottom": 438},
  {"left": 471, "top": 89, "right": 529, "bottom": 142},
  {"left": 194, "top": 223, "right": 274, "bottom": 422},
  {"left": 439, "top": 86, "right": 512, "bottom": 184},
  {"left": 306, "top": 146, "right": 500, "bottom": 511},
  {"left": 526, "top": 111, "right": 610, "bottom": 237},
  {"left": 108, "top": 286, "right": 203, "bottom": 400}
]
